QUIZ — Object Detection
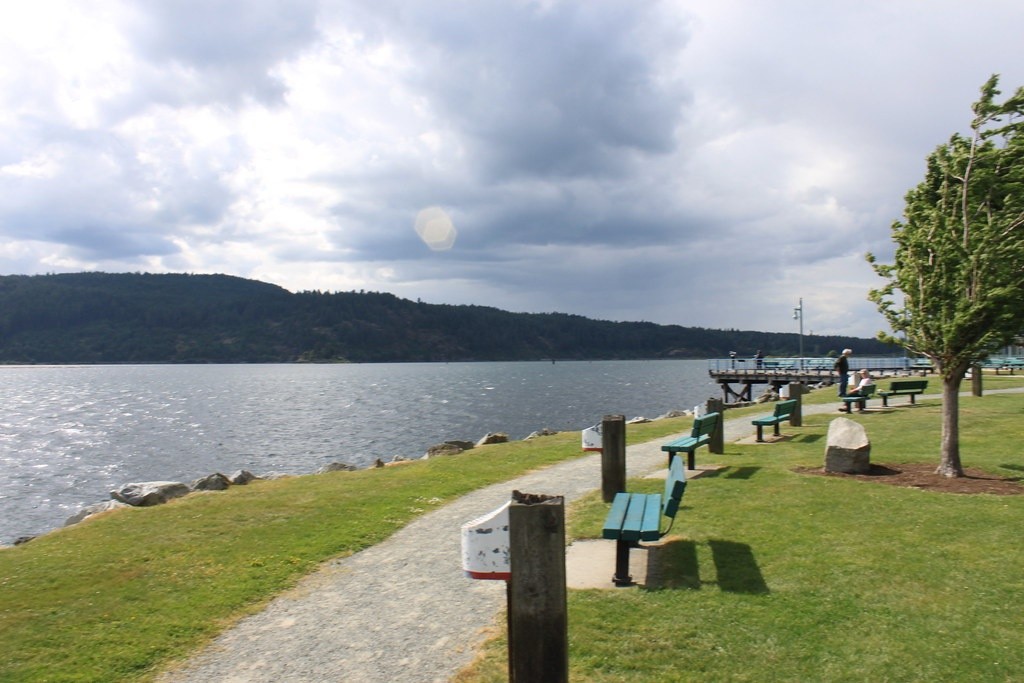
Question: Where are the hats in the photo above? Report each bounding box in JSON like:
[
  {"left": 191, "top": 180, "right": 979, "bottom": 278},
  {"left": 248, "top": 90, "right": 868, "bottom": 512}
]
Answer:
[
  {"left": 842, "top": 349, "right": 853, "bottom": 354},
  {"left": 858, "top": 368, "right": 868, "bottom": 374}
]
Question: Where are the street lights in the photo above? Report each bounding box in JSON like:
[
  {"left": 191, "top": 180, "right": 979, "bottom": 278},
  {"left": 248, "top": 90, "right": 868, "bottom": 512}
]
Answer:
[{"left": 791, "top": 298, "right": 804, "bottom": 369}]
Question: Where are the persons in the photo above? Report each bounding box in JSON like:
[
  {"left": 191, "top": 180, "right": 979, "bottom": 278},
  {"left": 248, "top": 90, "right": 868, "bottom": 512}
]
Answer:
[
  {"left": 839, "top": 369, "right": 872, "bottom": 412},
  {"left": 834, "top": 349, "right": 852, "bottom": 397},
  {"left": 755, "top": 350, "right": 763, "bottom": 369}
]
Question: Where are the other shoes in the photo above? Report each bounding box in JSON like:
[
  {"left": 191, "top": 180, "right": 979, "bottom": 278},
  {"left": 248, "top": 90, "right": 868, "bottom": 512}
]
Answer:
[{"left": 838, "top": 407, "right": 848, "bottom": 412}]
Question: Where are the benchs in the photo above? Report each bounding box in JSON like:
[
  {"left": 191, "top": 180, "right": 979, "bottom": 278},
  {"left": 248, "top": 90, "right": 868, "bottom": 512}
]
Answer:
[
  {"left": 751, "top": 398, "right": 798, "bottom": 443},
  {"left": 661, "top": 411, "right": 720, "bottom": 470},
  {"left": 981, "top": 360, "right": 1006, "bottom": 375},
  {"left": 763, "top": 361, "right": 782, "bottom": 371},
  {"left": 601, "top": 455, "right": 688, "bottom": 588},
  {"left": 877, "top": 380, "right": 928, "bottom": 407},
  {"left": 1007, "top": 361, "right": 1024, "bottom": 375},
  {"left": 843, "top": 384, "right": 876, "bottom": 415}
]
